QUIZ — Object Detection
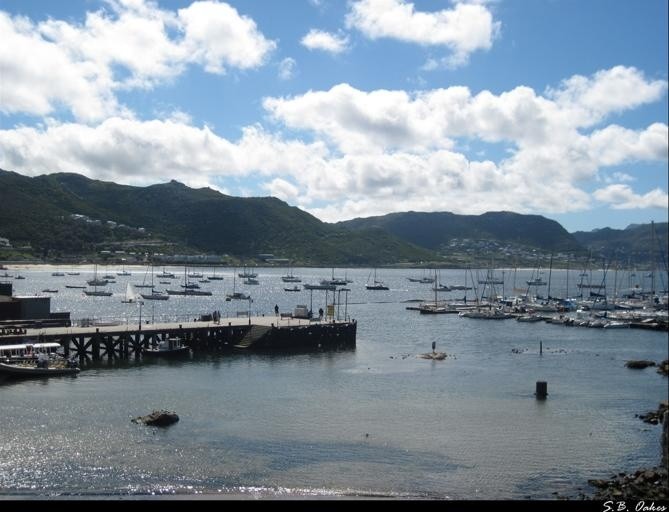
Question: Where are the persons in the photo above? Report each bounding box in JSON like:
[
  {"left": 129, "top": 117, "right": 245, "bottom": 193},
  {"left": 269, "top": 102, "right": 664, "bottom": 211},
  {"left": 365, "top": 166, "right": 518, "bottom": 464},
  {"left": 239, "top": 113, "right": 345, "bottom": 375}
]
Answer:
[
  {"left": 274, "top": 304, "right": 279, "bottom": 317},
  {"left": 212, "top": 311, "right": 221, "bottom": 326},
  {"left": 318, "top": 307, "right": 324, "bottom": 319}
]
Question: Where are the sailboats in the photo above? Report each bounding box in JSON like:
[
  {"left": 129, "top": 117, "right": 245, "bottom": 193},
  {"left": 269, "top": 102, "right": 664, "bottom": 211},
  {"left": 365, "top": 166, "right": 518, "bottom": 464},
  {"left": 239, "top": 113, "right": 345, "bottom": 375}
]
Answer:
[{"left": 0, "top": 260, "right": 669, "bottom": 331}]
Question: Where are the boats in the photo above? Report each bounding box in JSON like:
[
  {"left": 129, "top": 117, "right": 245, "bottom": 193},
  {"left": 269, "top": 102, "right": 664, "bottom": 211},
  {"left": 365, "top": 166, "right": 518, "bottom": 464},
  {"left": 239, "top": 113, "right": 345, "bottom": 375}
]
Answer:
[{"left": 0, "top": 342, "right": 81, "bottom": 376}]
